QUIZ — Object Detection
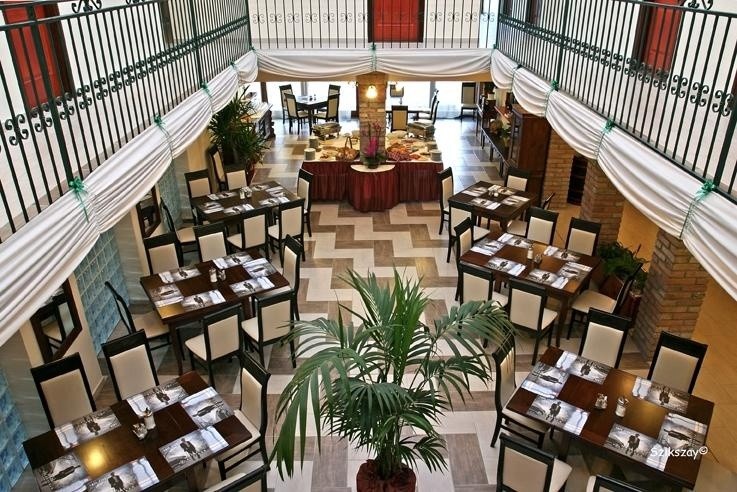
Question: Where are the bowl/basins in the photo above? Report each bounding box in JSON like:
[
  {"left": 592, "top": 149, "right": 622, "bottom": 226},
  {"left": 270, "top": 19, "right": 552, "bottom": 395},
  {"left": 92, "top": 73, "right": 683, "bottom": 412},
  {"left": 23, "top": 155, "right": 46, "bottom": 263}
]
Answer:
[
  {"left": 390, "top": 130, "right": 407, "bottom": 139},
  {"left": 350, "top": 139, "right": 357, "bottom": 145},
  {"left": 341, "top": 129, "right": 359, "bottom": 139}
]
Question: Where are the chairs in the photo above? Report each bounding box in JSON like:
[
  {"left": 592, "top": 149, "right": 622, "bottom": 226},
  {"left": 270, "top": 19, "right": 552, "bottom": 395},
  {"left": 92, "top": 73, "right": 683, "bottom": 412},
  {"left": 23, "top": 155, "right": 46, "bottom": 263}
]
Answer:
[
  {"left": 197, "top": 350, "right": 276, "bottom": 486},
  {"left": 226, "top": 212, "right": 271, "bottom": 264},
  {"left": 223, "top": 168, "right": 249, "bottom": 191},
  {"left": 101, "top": 327, "right": 162, "bottom": 404},
  {"left": 26, "top": 349, "right": 96, "bottom": 432},
  {"left": 494, "top": 430, "right": 573, "bottom": 492},
  {"left": 583, "top": 471, "right": 645, "bottom": 492},
  {"left": 564, "top": 213, "right": 601, "bottom": 257},
  {"left": 505, "top": 277, "right": 559, "bottom": 367},
  {"left": 240, "top": 285, "right": 299, "bottom": 371},
  {"left": 507, "top": 191, "right": 557, "bottom": 238},
  {"left": 266, "top": 205, "right": 307, "bottom": 268},
  {"left": 644, "top": 328, "right": 710, "bottom": 394},
  {"left": 460, "top": 82, "right": 479, "bottom": 123},
  {"left": 139, "top": 232, "right": 185, "bottom": 274},
  {"left": 454, "top": 262, "right": 511, "bottom": 348},
  {"left": 208, "top": 143, "right": 229, "bottom": 191},
  {"left": 293, "top": 167, "right": 315, "bottom": 238},
  {"left": 486, "top": 166, "right": 530, "bottom": 230},
  {"left": 104, "top": 81, "right": 343, "bottom": 392},
  {"left": 565, "top": 261, "right": 644, "bottom": 342},
  {"left": 181, "top": 167, "right": 210, "bottom": 198},
  {"left": 180, "top": 301, "right": 245, "bottom": 391},
  {"left": 279, "top": 232, "right": 306, "bottom": 328},
  {"left": 388, "top": 84, "right": 440, "bottom": 130},
  {"left": 104, "top": 280, "right": 186, "bottom": 365},
  {"left": 486, "top": 331, "right": 557, "bottom": 453},
  {"left": 434, "top": 166, "right": 455, "bottom": 234},
  {"left": 159, "top": 196, "right": 198, "bottom": 245},
  {"left": 197, "top": 462, "right": 272, "bottom": 492},
  {"left": 191, "top": 219, "right": 231, "bottom": 262},
  {"left": 573, "top": 305, "right": 635, "bottom": 370},
  {"left": 45, "top": 297, "right": 73, "bottom": 338},
  {"left": 525, "top": 205, "right": 560, "bottom": 246},
  {"left": 450, "top": 216, "right": 476, "bottom": 301},
  {"left": 277, "top": 82, "right": 342, "bottom": 138}
]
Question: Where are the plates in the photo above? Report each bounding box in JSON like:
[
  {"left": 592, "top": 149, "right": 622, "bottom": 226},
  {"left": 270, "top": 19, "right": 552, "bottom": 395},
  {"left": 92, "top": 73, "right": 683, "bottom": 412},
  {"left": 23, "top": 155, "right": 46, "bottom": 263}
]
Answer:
[
  {"left": 303, "top": 135, "right": 319, "bottom": 161},
  {"left": 424, "top": 140, "right": 442, "bottom": 161}
]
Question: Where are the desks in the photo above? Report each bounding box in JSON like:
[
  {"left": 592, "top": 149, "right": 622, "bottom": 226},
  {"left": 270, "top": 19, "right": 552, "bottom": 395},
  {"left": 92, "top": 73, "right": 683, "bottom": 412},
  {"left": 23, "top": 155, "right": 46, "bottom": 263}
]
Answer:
[
  {"left": 187, "top": 180, "right": 307, "bottom": 226},
  {"left": 454, "top": 228, "right": 604, "bottom": 341},
  {"left": 348, "top": 164, "right": 398, "bottom": 213},
  {"left": 136, "top": 248, "right": 293, "bottom": 364},
  {"left": 447, "top": 180, "right": 540, "bottom": 236},
  {"left": 17, "top": 368, "right": 264, "bottom": 492},
  {"left": 500, "top": 345, "right": 715, "bottom": 492},
  {"left": 298, "top": 128, "right": 445, "bottom": 205}
]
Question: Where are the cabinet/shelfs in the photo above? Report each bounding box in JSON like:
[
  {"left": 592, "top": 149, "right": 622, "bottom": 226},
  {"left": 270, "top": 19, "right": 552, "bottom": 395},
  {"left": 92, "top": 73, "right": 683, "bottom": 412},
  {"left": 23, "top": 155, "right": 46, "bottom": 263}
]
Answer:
[{"left": 474, "top": 89, "right": 553, "bottom": 194}]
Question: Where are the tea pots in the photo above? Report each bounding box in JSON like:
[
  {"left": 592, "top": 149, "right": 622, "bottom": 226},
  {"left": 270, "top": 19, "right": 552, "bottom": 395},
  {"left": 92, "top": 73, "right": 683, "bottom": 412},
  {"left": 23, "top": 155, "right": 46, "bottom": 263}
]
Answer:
[
  {"left": 533, "top": 253, "right": 543, "bottom": 264},
  {"left": 131, "top": 422, "right": 148, "bottom": 440},
  {"left": 216, "top": 269, "right": 226, "bottom": 282},
  {"left": 594, "top": 392, "right": 608, "bottom": 410},
  {"left": 244, "top": 187, "right": 253, "bottom": 199}
]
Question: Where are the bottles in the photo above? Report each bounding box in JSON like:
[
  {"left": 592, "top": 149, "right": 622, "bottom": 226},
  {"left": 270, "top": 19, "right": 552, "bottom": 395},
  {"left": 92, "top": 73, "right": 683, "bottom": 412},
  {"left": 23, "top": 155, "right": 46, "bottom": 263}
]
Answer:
[
  {"left": 614, "top": 395, "right": 629, "bottom": 418},
  {"left": 141, "top": 406, "right": 157, "bottom": 429},
  {"left": 239, "top": 186, "right": 245, "bottom": 202},
  {"left": 525, "top": 246, "right": 533, "bottom": 260},
  {"left": 487, "top": 187, "right": 499, "bottom": 199},
  {"left": 207, "top": 265, "right": 217, "bottom": 283},
  {"left": 308, "top": 93, "right": 316, "bottom": 103}
]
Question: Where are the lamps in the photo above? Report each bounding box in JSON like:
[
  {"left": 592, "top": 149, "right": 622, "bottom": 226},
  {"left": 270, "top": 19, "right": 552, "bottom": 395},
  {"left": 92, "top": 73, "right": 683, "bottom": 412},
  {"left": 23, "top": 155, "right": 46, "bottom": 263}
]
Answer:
[{"left": 365, "top": 83, "right": 378, "bottom": 99}]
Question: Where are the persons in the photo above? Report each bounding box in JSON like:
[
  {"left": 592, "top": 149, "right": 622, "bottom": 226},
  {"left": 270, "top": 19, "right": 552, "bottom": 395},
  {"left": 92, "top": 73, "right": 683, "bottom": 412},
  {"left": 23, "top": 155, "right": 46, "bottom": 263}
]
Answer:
[{"left": 43, "top": 185, "right": 703, "bottom": 492}]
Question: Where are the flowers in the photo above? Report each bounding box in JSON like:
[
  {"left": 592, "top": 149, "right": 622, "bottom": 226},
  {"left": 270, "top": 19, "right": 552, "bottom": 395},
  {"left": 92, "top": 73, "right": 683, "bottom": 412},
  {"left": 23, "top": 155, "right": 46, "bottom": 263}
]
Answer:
[{"left": 354, "top": 112, "right": 385, "bottom": 166}]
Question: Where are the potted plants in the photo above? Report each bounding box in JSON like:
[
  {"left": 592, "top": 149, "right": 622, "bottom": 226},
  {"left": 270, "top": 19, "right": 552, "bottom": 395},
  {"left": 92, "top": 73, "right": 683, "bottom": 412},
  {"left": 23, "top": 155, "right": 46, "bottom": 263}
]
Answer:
[{"left": 261, "top": 261, "right": 521, "bottom": 492}]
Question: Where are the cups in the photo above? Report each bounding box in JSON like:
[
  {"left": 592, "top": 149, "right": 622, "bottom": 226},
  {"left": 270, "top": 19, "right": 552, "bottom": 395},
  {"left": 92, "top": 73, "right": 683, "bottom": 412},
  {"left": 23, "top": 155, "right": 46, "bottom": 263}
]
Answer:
[
  {"left": 387, "top": 136, "right": 419, "bottom": 152},
  {"left": 487, "top": 93, "right": 494, "bottom": 100}
]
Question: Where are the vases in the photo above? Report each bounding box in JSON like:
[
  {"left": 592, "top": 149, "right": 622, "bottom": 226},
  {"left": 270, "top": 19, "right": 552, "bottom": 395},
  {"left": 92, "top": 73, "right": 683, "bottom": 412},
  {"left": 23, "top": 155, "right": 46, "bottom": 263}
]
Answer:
[{"left": 367, "top": 163, "right": 379, "bottom": 168}]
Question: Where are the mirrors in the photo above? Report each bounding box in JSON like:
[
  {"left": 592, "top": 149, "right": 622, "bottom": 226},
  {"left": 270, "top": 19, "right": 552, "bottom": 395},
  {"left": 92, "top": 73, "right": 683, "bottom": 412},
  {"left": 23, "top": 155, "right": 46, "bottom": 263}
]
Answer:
[{"left": 28, "top": 278, "right": 84, "bottom": 362}]
